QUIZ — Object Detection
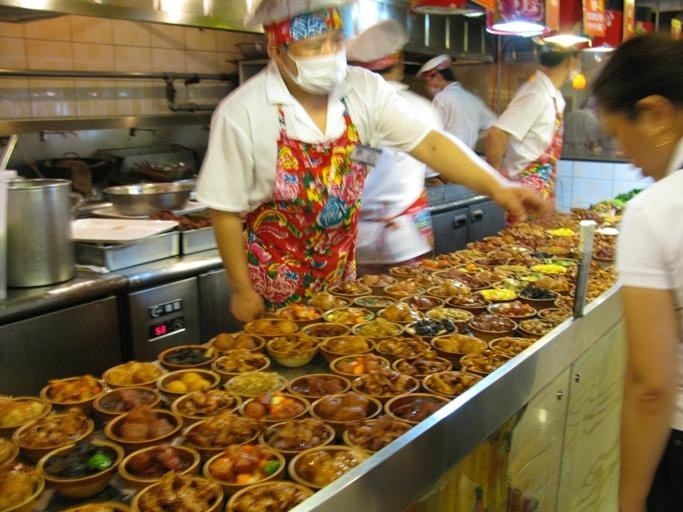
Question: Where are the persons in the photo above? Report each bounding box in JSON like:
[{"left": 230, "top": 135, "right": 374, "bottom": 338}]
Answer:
[
  {"left": 416, "top": 52, "right": 499, "bottom": 187},
  {"left": 590, "top": 32, "right": 683, "bottom": 512},
  {"left": 484, "top": 33, "right": 581, "bottom": 230},
  {"left": 344, "top": 18, "right": 436, "bottom": 279},
  {"left": 197, "top": 0, "right": 547, "bottom": 327}
]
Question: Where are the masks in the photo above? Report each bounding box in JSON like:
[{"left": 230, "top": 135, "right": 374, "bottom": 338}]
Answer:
[{"left": 279, "top": 50, "right": 346, "bottom": 96}]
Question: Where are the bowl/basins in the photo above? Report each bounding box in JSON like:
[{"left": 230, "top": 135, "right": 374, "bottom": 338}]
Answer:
[
  {"left": 234, "top": 40, "right": 267, "bottom": 60},
  {"left": 101, "top": 183, "right": 194, "bottom": 215},
  {"left": 0, "top": 203, "right": 624, "bottom": 512}
]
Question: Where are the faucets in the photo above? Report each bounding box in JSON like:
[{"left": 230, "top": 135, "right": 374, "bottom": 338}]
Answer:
[
  {"left": 39, "top": 127, "right": 68, "bottom": 143},
  {"left": 128, "top": 124, "right": 160, "bottom": 139}
]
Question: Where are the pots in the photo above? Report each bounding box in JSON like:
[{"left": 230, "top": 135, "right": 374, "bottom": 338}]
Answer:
[
  {"left": 7, "top": 179, "right": 85, "bottom": 288},
  {"left": 32, "top": 152, "right": 111, "bottom": 187}
]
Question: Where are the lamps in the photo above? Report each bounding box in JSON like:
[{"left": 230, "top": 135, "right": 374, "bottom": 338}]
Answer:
[{"left": 409, "top": 0, "right": 623, "bottom": 53}]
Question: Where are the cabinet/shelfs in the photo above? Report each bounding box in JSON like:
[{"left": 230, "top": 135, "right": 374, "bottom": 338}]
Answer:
[{"left": 430, "top": 193, "right": 508, "bottom": 254}]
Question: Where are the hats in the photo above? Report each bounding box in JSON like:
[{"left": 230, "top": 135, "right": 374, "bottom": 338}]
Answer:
[
  {"left": 341, "top": 20, "right": 411, "bottom": 72},
  {"left": 244, "top": 1, "right": 357, "bottom": 43},
  {"left": 413, "top": 53, "right": 455, "bottom": 80}
]
